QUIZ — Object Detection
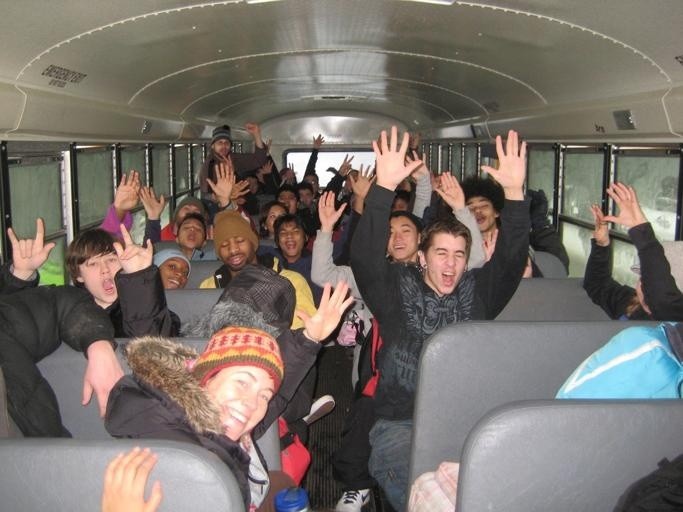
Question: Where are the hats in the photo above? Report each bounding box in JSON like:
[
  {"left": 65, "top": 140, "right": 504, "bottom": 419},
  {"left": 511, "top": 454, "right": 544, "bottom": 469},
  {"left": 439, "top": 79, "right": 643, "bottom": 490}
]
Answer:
[
  {"left": 213, "top": 210, "right": 258, "bottom": 258},
  {"left": 189, "top": 301, "right": 284, "bottom": 394},
  {"left": 153, "top": 249, "right": 191, "bottom": 278},
  {"left": 211, "top": 125, "right": 232, "bottom": 145}
]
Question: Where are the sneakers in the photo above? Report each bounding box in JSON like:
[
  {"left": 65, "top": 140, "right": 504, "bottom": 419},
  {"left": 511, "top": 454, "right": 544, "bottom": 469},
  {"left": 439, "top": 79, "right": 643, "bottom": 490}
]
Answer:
[
  {"left": 302, "top": 395, "right": 335, "bottom": 424},
  {"left": 335, "top": 488, "right": 370, "bottom": 512}
]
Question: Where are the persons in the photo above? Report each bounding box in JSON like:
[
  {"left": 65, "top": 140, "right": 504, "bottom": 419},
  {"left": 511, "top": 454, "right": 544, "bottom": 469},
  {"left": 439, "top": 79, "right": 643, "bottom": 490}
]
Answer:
[{"left": 1, "top": 114, "right": 683, "bottom": 512}]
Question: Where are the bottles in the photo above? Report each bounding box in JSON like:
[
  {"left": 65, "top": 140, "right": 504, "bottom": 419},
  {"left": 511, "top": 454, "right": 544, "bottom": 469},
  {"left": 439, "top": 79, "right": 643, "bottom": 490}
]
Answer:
[{"left": 273, "top": 486, "right": 312, "bottom": 512}]
